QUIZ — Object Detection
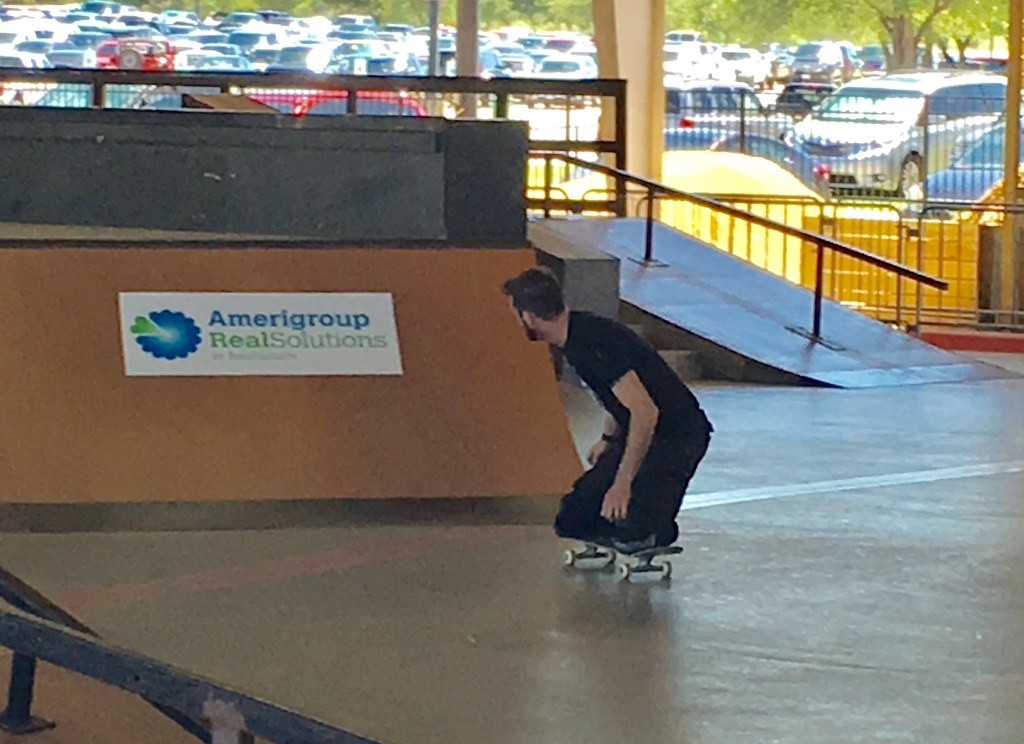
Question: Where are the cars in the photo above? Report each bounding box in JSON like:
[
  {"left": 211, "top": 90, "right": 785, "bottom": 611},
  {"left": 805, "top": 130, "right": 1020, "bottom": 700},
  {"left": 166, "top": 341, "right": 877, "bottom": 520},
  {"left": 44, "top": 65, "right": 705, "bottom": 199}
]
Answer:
[
  {"left": 903, "top": 118, "right": 1024, "bottom": 221},
  {"left": 0, "top": 0, "right": 1007, "bottom": 200}
]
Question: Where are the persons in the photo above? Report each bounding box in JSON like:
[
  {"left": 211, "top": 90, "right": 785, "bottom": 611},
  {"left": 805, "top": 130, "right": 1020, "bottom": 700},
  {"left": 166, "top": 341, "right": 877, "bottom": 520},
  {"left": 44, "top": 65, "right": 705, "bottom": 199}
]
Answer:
[{"left": 501, "top": 265, "right": 713, "bottom": 555}]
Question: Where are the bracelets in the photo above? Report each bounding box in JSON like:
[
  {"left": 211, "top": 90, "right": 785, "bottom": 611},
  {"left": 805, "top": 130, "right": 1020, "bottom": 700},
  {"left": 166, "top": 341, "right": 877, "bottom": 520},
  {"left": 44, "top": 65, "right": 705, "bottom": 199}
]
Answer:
[{"left": 602, "top": 433, "right": 622, "bottom": 445}]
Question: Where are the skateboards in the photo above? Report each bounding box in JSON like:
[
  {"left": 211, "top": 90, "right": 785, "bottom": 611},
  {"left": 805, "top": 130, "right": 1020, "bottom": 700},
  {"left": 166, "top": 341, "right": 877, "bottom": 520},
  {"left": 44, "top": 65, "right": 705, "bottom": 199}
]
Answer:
[{"left": 552, "top": 525, "right": 684, "bottom": 580}]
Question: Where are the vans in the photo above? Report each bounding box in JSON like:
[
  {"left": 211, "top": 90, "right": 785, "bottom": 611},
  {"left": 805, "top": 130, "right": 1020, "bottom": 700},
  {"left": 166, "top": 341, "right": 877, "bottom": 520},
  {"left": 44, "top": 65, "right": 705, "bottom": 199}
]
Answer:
[{"left": 780, "top": 64, "right": 1008, "bottom": 198}]
